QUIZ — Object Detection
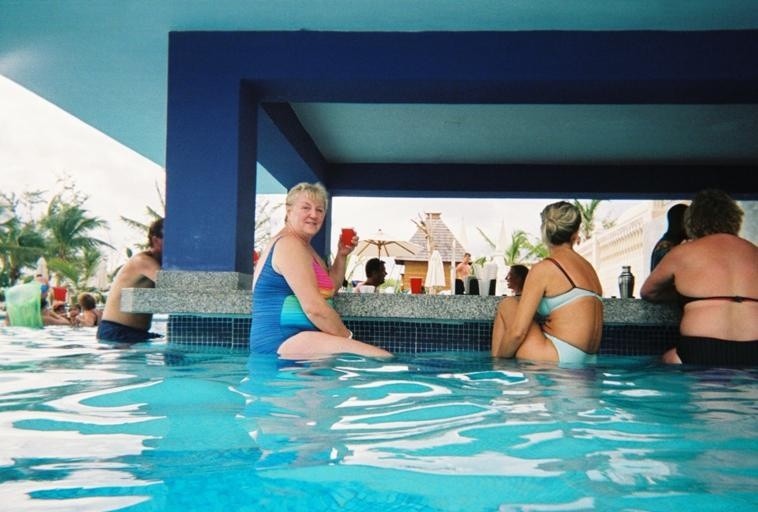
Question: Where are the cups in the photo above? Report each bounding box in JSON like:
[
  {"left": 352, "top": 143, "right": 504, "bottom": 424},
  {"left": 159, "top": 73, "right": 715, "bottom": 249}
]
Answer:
[
  {"left": 410, "top": 277, "right": 423, "bottom": 294},
  {"left": 341, "top": 228, "right": 355, "bottom": 247}
]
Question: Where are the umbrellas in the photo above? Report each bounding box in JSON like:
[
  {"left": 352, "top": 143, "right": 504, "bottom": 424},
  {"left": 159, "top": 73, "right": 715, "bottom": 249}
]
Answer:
[
  {"left": 354, "top": 228, "right": 425, "bottom": 260},
  {"left": 423, "top": 247, "right": 447, "bottom": 294}
]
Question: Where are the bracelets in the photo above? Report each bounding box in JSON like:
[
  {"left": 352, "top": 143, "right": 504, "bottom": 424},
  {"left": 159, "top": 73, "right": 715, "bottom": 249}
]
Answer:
[{"left": 348, "top": 329, "right": 353, "bottom": 339}]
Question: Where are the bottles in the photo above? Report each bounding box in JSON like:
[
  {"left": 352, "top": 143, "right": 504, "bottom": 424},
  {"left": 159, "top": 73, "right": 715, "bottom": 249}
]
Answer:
[{"left": 618, "top": 266, "right": 634, "bottom": 299}]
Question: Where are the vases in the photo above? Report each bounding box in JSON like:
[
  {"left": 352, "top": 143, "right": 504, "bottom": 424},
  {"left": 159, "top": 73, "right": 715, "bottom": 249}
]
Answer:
[{"left": 617, "top": 266, "right": 635, "bottom": 298}]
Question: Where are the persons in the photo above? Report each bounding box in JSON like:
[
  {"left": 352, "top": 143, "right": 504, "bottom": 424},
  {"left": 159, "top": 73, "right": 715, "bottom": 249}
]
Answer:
[
  {"left": 95, "top": 216, "right": 165, "bottom": 344},
  {"left": 457, "top": 252, "right": 474, "bottom": 282},
  {"left": 505, "top": 264, "right": 529, "bottom": 296},
  {"left": 650, "top": 203, "right": 691, "bottom": 273},
  {"left": 247, "top": 180, "right": 395, "bottom": 363},
  {"left": 492, "top": 201, "right": 604, "bottom": 365},
  {"left": 352, "top": 257, "right": 389, "bottom": 293},
  {"left": 1, "top": 271, "right": 103, "bottom": 328},
  {"left": 638, "top": 186, "right": 757, "bottom": 369}
]
